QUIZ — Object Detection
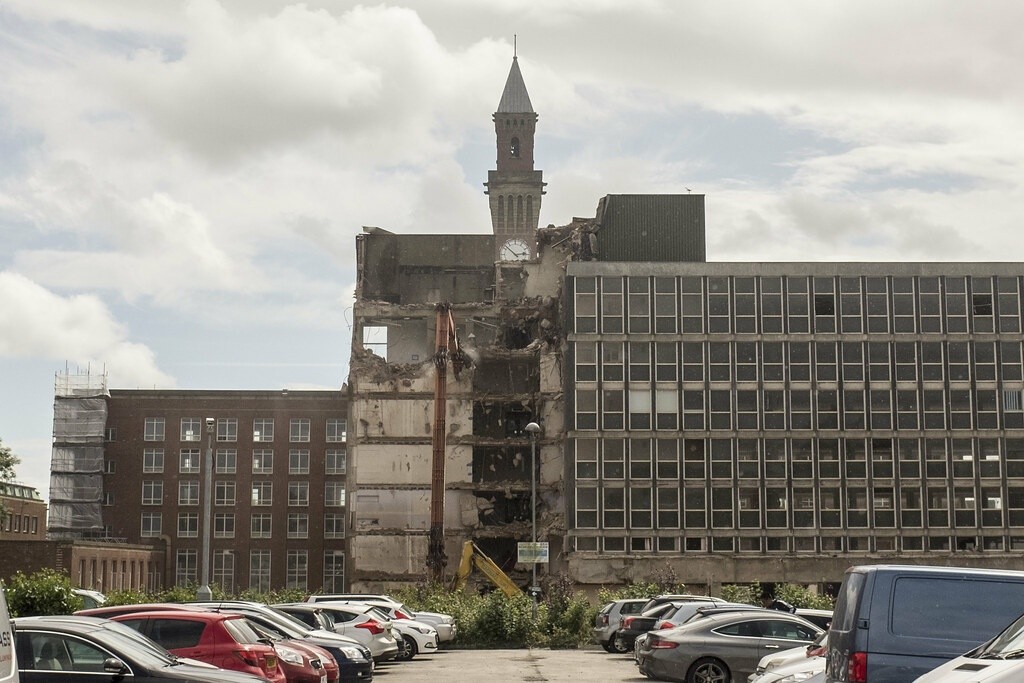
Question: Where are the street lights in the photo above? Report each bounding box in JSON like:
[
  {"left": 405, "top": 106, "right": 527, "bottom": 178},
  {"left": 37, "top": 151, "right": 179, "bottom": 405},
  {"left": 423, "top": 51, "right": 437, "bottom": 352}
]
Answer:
[{"left": 525, "top": 422, "right": 543, "bottom": 621}]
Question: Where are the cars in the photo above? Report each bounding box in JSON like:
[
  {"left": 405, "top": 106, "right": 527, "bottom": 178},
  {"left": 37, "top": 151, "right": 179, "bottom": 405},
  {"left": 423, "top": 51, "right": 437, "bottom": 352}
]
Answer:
[{"left": 0, "top": 578, "right": 457, "bottom": 683}]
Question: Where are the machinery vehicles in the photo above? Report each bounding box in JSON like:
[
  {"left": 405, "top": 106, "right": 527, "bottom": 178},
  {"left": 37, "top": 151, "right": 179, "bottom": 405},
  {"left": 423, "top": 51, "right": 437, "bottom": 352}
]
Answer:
[{"left": 445, "top": 540, "right": 533, "bottom": 615}]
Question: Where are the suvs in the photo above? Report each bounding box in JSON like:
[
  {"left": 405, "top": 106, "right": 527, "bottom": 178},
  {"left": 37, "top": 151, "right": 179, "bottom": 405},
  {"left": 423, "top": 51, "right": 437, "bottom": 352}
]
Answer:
[{"left": 591, "top": 564, "right": 1024, "bottom": 683}]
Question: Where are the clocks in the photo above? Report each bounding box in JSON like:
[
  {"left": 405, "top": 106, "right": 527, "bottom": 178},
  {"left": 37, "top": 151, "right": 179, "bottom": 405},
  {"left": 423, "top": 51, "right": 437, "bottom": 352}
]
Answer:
[{"left": 499, "top": 238, "right": 533, "bottom": 262}]
